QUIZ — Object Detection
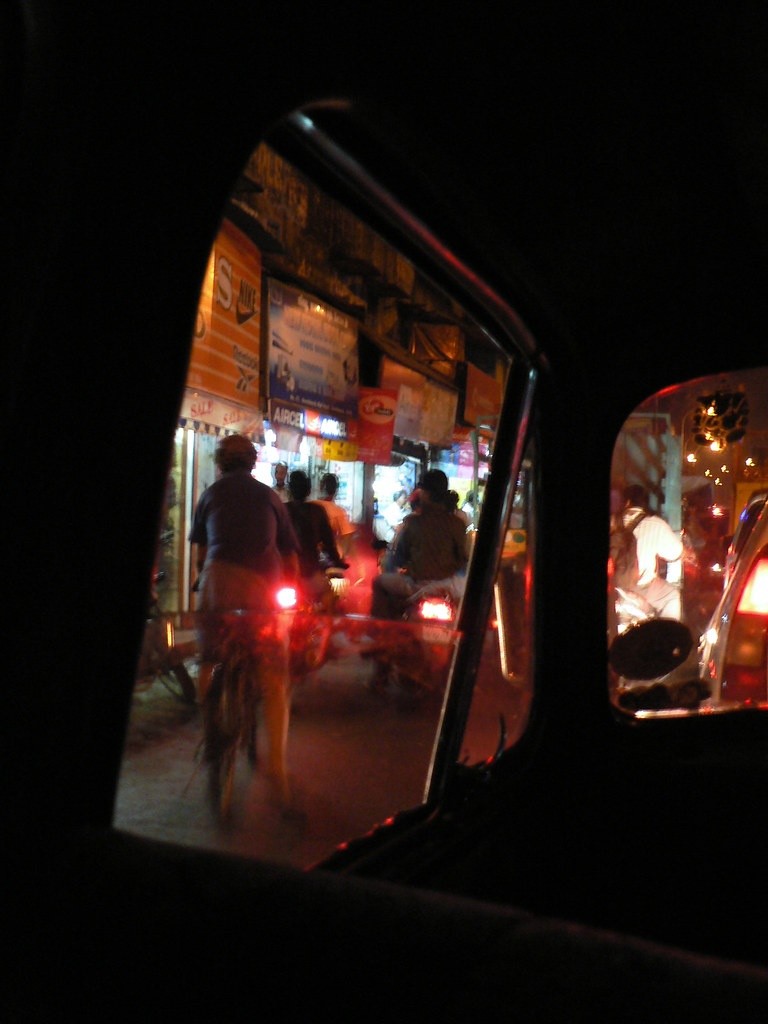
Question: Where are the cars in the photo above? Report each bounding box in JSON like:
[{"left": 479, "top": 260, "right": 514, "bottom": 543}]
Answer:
[{"left": 690, "top": 499, "right": 768, "bottom": 713}]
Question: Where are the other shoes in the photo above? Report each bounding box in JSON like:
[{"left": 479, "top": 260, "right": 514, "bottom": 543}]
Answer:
[{"left": 278, "top": 809, "right": 308, "bottom": 823}]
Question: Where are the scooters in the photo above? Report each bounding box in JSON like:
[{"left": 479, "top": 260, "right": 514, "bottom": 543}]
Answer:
[
  {"left": 136, "top": 572, "right": 197, "bottom": 703},
  {"left": 281, "top": 554, "right": 352, "bottom": 678},
  {"left": 614, "top": 588, "right": 677, "bottom": 694},
  {"left": 361, "top": 538, "right": 460, "bottom": 712}
]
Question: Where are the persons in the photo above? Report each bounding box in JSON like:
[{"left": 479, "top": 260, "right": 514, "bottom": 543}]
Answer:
[
  {"left": 189, "top": 433, "right": 299, "bottom": 814},
  {"left": 273, "top": 456, "right": 360, "bottom": 609},
  {"left": 610, "top": 482, "right": 685, "bottom": 619},
  {"left": 366, "top": 466, "right": 471, "bottom": 692}
]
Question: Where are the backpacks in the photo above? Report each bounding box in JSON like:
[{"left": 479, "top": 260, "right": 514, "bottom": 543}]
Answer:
[{"left": 609, "top": 512, "right": 653, "bottom": 585}]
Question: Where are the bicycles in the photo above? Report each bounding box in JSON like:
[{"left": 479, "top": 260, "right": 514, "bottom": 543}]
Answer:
[{"left": 191, "top": 583, "right": 273, "bottom": 821}]
